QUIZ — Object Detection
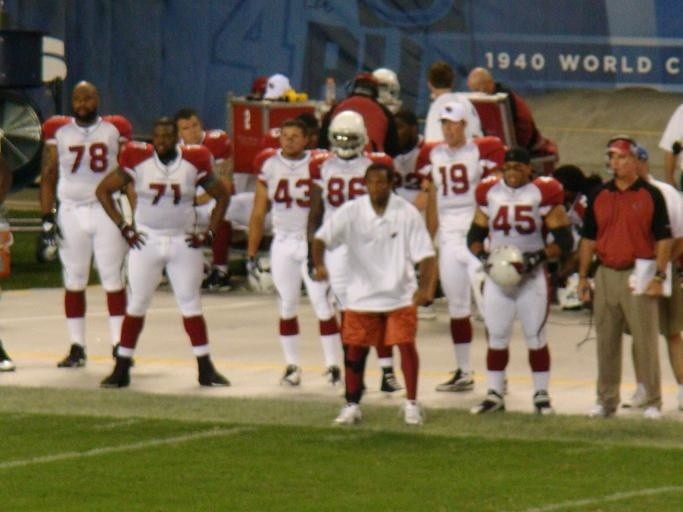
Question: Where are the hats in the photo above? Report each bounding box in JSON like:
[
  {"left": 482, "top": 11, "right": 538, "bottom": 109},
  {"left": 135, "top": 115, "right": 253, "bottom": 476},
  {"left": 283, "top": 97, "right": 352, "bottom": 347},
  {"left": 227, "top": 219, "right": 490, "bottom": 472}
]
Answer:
[
  {"left": 247, "top": 78, "right": 267, "bottom": 100},
  {"left": 440, "top": 104, "right": 466, "bottom": 123},
  {"left": 504, "top": 149, "right": 530, "bottom": 164},
  {"left": 264, "top": 76, "right": 289, "bottom": 100},
  {"left": 605, "top": 137, "right": 638, "bottom": 157}
]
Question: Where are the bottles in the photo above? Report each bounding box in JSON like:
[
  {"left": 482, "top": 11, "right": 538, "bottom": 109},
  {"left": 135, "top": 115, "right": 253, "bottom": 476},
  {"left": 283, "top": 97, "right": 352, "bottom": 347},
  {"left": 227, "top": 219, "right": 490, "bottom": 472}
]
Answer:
[{"left": 326, "top": 77, "right": 336, "bottom": 106}]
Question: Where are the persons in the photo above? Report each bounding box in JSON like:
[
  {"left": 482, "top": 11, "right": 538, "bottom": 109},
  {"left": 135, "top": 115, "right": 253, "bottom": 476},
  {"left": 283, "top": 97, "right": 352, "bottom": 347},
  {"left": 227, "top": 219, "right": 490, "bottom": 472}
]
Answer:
[
  {"left": 245, "top": 119, "right": 343, "bottom": 387},
  {"left": 661, "top": 93, "right": 683, "bottom": 188},
  {"left": 0, "top": 159, "right": 15, "bottom": 372},
  {"left": 40, "top": 80, "right": 133, "bottom": 368},
  {"left": 374, "top": 66, "right": 420, "bottom": 146},
  {"left": 308, "top": 109, "right": 408, "bottom": 395},
  {"left": 467, "top": 67, "right": 558, "bottom": 172},
  {"left": 635, "top": 145, "right": 683, "bottom": 422},
  {"left": 577, "top": 135, "right": 674, "bottom": 422},
  {"left": 426, "top": 62, "right": 485, "bottom": 147},
  {"left": 467, "top": 148, "right": 573, "bottom": 416},
  {"left": 312, "top": 164, "right": 436, "bottom": 424},
  {"left": 317, "top": 72, "right": 404, "bottom": 156},
  {"left": 416, "top": 104, "right": 511, "bottom": 394},
  {"left": 174, "top": 109, "right": 235, "bottom": 294},
  {"left": 95, "top": 118, "right": 230, "bottom": 389}
]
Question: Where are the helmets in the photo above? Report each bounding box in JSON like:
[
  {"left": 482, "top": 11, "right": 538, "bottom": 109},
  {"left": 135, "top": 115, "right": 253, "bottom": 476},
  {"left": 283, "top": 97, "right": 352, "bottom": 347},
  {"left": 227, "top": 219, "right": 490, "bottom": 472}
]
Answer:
[
  {"left": 330, "top": 111, "right": 368, "bottom": 157},
  {"left": 558, "top": 273, "right": 590, "bottom": 308},
  {"left": 374, "top": 69, "right": 399, "bottom": 99},
  {"left": 249, "top": 260, "right": 275, "bottom": 292},
  {"left": 486, "top": 244, "right": 524, "bottom": 286}
]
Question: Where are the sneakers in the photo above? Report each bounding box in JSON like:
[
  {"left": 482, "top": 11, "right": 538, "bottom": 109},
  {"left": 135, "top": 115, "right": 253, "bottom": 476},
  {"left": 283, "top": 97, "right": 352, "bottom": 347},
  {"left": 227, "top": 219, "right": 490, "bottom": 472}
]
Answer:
[
  {"left": 624, "top": 389, "right": 648, "bottom": 408},
  {"left": 333, "top": 405, "right": 362, "bottom": 426},
  {"left": 471, "top": 394, "right": 504, "bottom": 413},
  {"left": 381, "top": 374, "right": 406, "bottom": 395},
  {"left": 0, "top": 348, "right": 14, "bottom": 370},
  {"left": 404, "top": 401, "right": 421, "bottom": 424},
  {"left": 199, "top": 369, "right": 230, "bottom": 386},
  {"left": 199, "top": 269, "right": 230, "bottom": 291},
  {"left": 323, "top": 367, "right": 344, "bottom": 389},
  {"left": 534, "top": 390, "right": 552, "bottom": 416},
  {"left": 101, "top": 368, "right": 130, "bottom": 387},
  {"left": 58, "top": 346, "right": 85, "bottom": 367},
  {"left": 279, "top": 364, "right": 301, "bottom": 387},
  {"left": 435, "top": 369, "right": 475, "bottom": 391}
]
